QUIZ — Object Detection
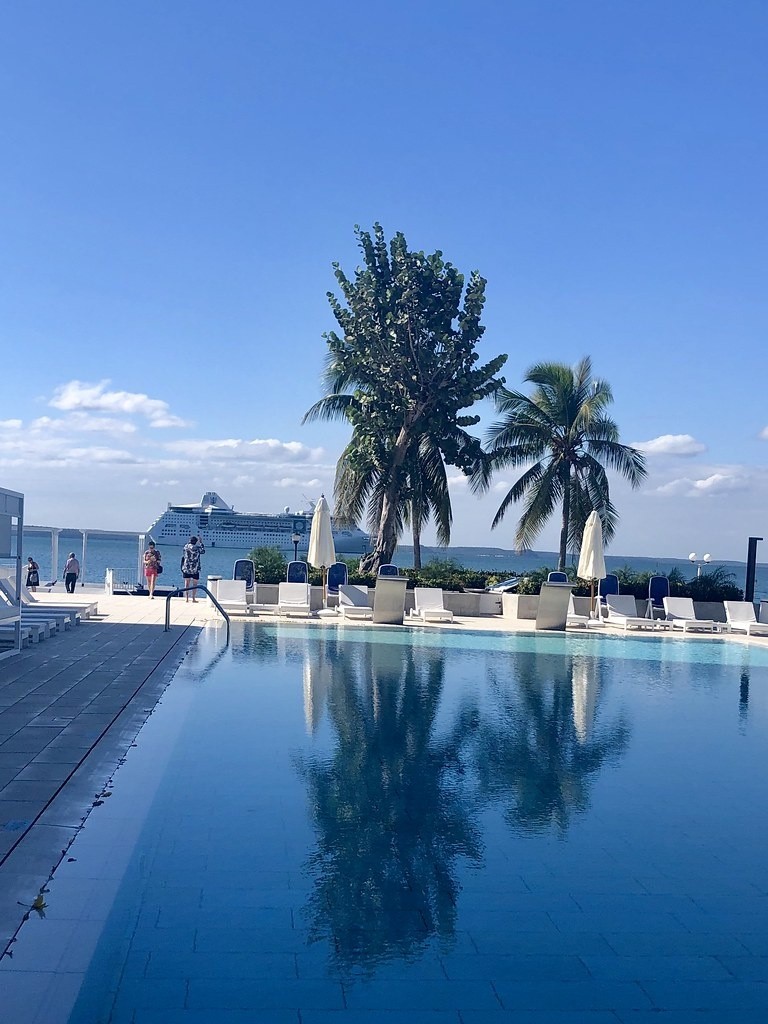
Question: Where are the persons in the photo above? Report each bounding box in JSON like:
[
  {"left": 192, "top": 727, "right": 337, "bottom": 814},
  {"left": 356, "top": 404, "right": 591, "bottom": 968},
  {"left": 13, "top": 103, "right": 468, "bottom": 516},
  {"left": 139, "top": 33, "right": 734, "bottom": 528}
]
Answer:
[
  {"left": 144, "top": 541, "right": 161, "bottom": 599},
  {"left": 62, "top": 552, "right": 80, "bottom": 593},
  {"left": 181, "top": 537, "right": 205, "bottom": 603},
  {"left": 25, "top": 556, "right": 39, "bottom": 592}
]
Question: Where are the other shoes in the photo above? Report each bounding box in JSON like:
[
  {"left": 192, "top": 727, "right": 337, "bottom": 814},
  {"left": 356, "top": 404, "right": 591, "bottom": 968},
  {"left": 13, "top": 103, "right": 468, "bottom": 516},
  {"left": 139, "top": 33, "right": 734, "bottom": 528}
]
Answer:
[
  {"left": 148, "top": 595, "right": 150, "bottom": 598},
  {"left": 150, "top": 595, "right": 154, "bottom": 599},
  {"left": 67, "top": 588, "right": 70, "bottom": 593},
  {"left": 185, "top": 600, "right": 189, "bottom": 602},
  {"left": 70, "top": 591, "right": 74, "bottom": 593},
  {"left": 192, "top": 600, "right": 198, "bottom": 602}
]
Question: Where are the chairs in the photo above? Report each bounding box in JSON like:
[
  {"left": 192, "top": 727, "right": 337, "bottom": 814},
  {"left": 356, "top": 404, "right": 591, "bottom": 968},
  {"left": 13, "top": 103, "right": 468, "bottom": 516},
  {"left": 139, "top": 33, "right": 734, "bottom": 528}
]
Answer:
[
  {"left": 324, "top": 561, "right": 348, "bottom": 610},
  {"left": 662, "top": 595, "right": 713, "bottom": 635},
  {"left": 335, "top": 584, "right": 374, "bottom": 620},
  {"left": 410, "top": 587, "right": 453, "bottom": 623},
  {"left": 214, "top": 580, "right": 249, "bottom": 617},
  {"left": 605, "top": 593, "right": 659, "bottom": 632},
  {"left": 547, "top": 571, "right": 569, "bottom": 582},
  {"left": 724, "top": 601, "right": 768, "bottom": 636},
  {"left": 286, "top": 561, "right": 308, "bottom": 583},
  {"left": 644, "top": 575, "right": 670, "bottom": 620},
  {"left": 233, "top": 559, "right": 257, "bottom": 606},
  {"left": 378, "top": 563, "right": 399, "bottom": 576},
  {"left": 0, "top": 575, "right": 99, "bottom": 651},
  {"left": 277, "top": 582, "right": 311, "bottom": 619},
  {"left": 594, "top": 574, "right": 619, "bottom": 621}
]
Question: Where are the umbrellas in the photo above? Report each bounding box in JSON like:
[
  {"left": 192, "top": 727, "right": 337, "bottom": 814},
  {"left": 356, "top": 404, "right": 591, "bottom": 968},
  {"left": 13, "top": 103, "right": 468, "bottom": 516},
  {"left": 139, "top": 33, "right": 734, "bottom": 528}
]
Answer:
[
  {"left": 577, "top": 508, "right": 607, "bottom": 626},
  {"left": 308, "top": 492, "right": 337, "bottom": 617}
]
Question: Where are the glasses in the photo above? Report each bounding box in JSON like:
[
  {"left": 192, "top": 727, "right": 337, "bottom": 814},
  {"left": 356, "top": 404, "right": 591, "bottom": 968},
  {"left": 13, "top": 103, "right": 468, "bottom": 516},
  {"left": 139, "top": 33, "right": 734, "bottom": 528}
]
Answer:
[{"left": 28, "top": 560, "right": 32, "bottom": 561}]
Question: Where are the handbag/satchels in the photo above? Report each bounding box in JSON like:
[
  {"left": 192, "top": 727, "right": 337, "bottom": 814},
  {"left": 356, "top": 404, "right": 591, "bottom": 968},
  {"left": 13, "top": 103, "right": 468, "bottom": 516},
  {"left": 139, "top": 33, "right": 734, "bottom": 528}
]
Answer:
[
  {"left": 157, "top": 565, "right": 163, "bottom": 574},
  {"left": 29, "top": 573, "right": 38, "bottom": 582}
]
